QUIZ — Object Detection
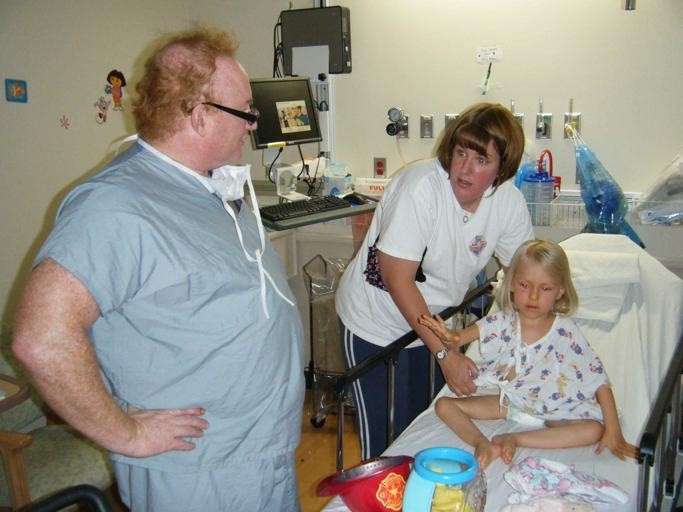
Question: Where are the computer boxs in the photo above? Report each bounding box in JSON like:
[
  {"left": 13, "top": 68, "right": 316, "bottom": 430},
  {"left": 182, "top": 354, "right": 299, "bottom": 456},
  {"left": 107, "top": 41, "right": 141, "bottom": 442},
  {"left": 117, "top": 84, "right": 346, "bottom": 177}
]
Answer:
[{"left": 281, "top": 6, "right": 352, "bottom": 75}]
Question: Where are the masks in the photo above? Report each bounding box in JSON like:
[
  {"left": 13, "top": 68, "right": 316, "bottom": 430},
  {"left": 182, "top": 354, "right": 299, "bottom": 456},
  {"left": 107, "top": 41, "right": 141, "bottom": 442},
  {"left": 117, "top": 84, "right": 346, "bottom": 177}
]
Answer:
[{"left": 200, "top": 164, "right": 251, "bottom": 201}]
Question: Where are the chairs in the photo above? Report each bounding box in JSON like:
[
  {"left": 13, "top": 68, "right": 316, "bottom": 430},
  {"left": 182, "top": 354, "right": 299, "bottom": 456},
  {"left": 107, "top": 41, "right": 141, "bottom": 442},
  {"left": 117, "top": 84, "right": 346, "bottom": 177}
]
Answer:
[{"left": 0, "top": 350, "right": 117, "bottom": 512}]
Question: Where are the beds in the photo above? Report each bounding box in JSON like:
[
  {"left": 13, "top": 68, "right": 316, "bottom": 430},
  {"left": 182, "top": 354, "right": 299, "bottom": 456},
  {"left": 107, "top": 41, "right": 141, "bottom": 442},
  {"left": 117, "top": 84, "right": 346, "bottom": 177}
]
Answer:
[{"left": 318, "top": 232, "right": 683, "bottom": 512}]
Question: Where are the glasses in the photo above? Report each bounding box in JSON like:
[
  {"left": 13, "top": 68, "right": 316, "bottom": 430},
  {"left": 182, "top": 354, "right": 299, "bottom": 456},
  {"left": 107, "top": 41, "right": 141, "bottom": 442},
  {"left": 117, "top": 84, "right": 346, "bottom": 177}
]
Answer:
[{"left": 187, "top": 101, "right": 260, "bottom": 127}]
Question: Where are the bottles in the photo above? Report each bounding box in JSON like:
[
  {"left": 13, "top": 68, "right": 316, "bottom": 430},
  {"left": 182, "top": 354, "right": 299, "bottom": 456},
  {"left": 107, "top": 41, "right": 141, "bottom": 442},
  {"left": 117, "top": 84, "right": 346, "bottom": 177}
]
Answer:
[
  {"left": 523, "top": 171, "right": 561, "bottom": 225},
  {"left": 320, "top": 150, "right": 331, "bottom": 197},
  {"left": 402, "top": 446, "right": 487, "bottom": 512}
]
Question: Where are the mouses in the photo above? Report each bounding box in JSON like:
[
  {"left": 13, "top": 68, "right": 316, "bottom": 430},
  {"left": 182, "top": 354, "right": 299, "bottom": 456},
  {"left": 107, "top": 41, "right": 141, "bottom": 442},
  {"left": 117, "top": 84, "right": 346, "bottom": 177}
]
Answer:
[{"left": 344, "top": 193, "right": 366, "bottom": 204}]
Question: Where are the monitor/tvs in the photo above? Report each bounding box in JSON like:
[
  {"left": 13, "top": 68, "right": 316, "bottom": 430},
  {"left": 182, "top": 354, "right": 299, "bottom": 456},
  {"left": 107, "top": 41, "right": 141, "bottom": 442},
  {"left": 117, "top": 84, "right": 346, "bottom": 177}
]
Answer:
[{"left": 249, "top": 77, "right": 323, "bottom": 150}]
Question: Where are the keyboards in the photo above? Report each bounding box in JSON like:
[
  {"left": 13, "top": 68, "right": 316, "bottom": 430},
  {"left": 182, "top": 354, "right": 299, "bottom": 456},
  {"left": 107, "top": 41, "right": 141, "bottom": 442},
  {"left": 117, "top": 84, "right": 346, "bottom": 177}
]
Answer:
[{"left": 260, "top": 194, "right": 352, "bottom": 232}]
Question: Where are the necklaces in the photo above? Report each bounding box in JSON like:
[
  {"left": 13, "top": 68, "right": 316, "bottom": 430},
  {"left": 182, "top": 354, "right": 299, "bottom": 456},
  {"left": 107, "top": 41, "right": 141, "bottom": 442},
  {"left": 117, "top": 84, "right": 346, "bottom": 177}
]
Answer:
[{"left": 462, "top": 202, "right": 476, "bottom": 223}]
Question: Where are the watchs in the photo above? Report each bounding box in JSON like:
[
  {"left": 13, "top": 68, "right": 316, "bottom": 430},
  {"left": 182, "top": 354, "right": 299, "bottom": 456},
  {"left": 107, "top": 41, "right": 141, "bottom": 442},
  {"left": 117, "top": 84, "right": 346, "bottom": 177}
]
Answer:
[{"left": 435, "top": 346, "right": 450, "bottom": 364}]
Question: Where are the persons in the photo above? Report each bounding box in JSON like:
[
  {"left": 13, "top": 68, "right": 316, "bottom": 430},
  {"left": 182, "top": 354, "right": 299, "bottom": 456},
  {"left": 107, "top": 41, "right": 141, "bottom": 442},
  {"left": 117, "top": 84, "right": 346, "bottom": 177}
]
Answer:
[
  {"left": 281, "top": 106, "right": 309, "bottom": 128},
  {"left": 334, "top": 102, "right": 535, "bottom": 463},
  {"left": 419, "top": 237, "right": 641, "bottom": 471},
  {"left": 105, "top": 69, "right": 127, "bottom": 111},
  {"left": 10, "top": 28, "right": 306, "bottom": 512}
]
